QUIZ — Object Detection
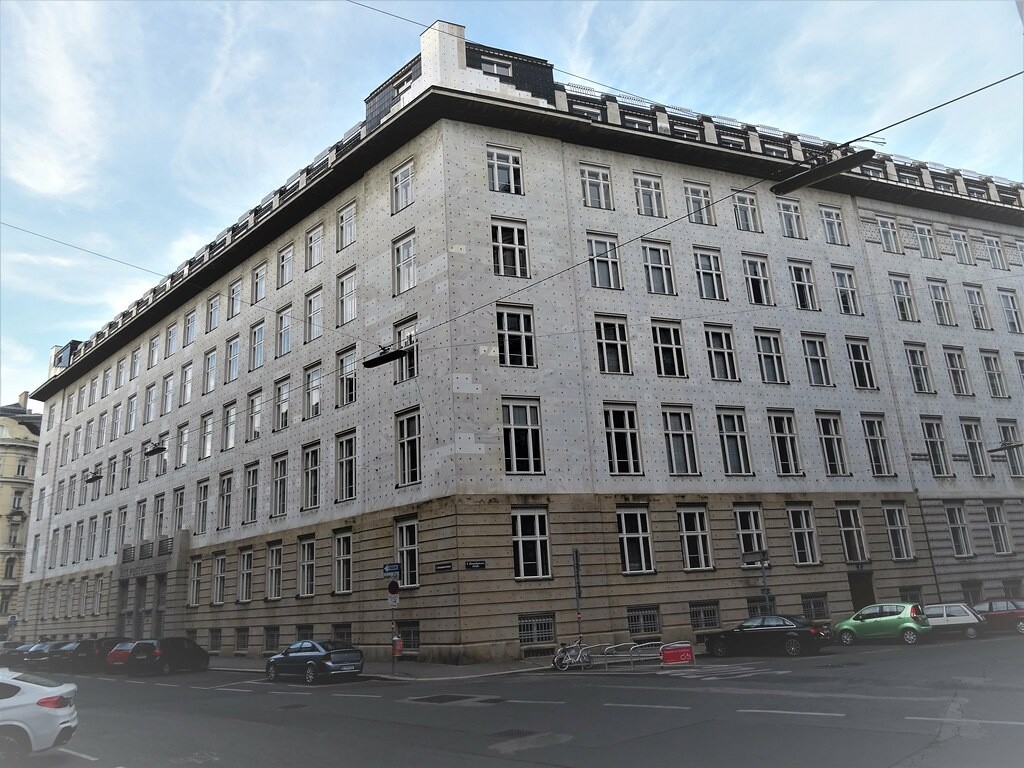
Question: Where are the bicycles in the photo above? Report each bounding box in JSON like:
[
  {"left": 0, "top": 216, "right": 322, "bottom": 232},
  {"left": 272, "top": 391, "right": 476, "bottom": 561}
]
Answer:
[{"left": 551, "top": 635, "right": 594, "bottom": 671}]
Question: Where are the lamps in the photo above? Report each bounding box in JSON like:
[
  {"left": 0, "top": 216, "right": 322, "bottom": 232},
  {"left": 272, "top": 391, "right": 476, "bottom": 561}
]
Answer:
[
  {"left": 85, "top": 471, "right": 102, "bottom": 483},
  {"left": 144, "top": 442, "right": 167, "bottom": 456},
  {"left": 770, "top": 149, "right": 875, "bottom": 196},
  {"left": 362, "top": 346, "right": 408, "bottom": 368}
]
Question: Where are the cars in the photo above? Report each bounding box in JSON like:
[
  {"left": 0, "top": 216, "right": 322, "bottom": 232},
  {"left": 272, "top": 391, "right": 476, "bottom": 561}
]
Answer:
[
  {"left": 923, "top": 602, "right": 991, "bottom": 639},
  {"left": 1, "top": 635, "right": 211, "bottom": 677},
  {"left": 0, "top": 665, "right": 77, "bottom": 767},
  {"left": 264, "top": 639, "right": 364, "bottom": 685},
  {"left": 972, "top": 598, "right": 1024, "bottom": 635},
  {"left": 705, "top": 613, "right": 834, "bottom": 658},
  {"left": 833, "top": 602, "right": 933, "bottom": 647}
]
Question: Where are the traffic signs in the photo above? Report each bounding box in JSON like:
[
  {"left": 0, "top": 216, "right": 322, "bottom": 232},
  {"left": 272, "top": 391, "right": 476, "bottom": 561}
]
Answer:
[{"left": 382, "top": 562, "right": 399, "bottom": 573}]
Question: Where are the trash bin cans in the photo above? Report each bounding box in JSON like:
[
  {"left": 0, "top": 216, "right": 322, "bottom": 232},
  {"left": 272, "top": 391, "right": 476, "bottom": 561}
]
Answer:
[{"left": 391, "top": 634, "right": 404, "bottom": 657}]
